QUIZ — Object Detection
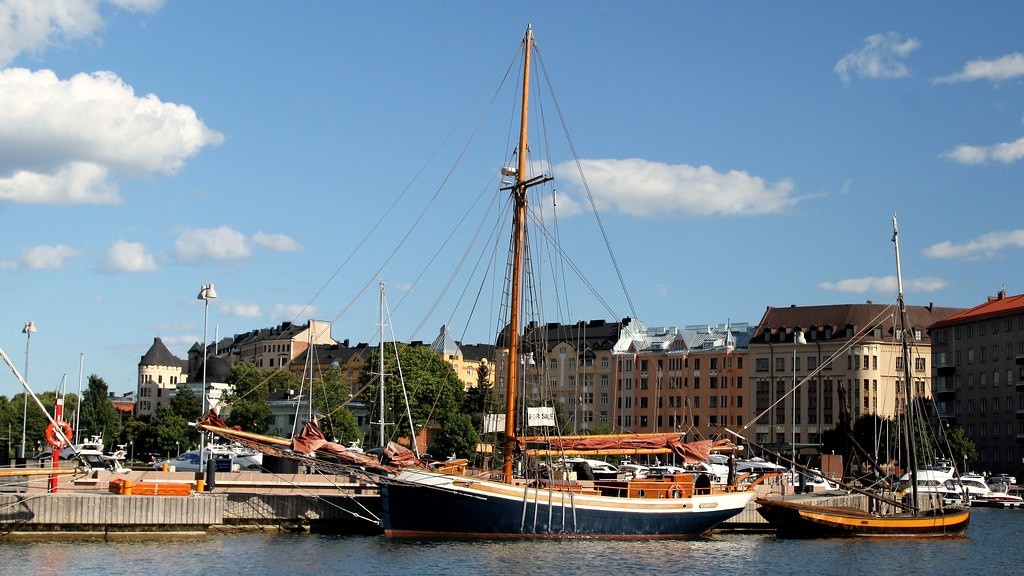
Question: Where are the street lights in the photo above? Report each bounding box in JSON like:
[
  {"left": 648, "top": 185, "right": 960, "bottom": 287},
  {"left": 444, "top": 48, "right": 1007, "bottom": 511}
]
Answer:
[
  {"left": 20, "top": 320, "right": 39, "bottom": 464},
  {"left": 197, "top": 282, "right": 218, "bottom": 469}
]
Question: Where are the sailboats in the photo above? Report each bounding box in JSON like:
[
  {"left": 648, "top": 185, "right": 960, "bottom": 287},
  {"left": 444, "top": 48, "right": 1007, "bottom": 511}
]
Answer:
[
  {"left": 755, "top": 213, "right": 973, "bottom": 537},
  {"left": 188, "top": 23, "right": 754, "bottom": 539}
]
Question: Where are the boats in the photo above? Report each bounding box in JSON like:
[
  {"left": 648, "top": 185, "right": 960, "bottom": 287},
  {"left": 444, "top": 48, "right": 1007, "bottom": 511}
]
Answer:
[
  {"left": 151, "top": 441, "right": 263, "bottom": 472},
  {"left": 896, "top": 455, "right": 1024, "bottom": 508},
  {"left": 547, "top": 452, "right": 841, "bottom": 493},
  {"left": 36, "top": 432, "right": 133, "bottom": 474}
]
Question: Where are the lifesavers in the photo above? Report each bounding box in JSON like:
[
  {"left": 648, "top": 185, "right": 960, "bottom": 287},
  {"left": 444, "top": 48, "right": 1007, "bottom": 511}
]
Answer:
[{"left": 46, "top": 422, "right": 73, "bottom": 449}]
